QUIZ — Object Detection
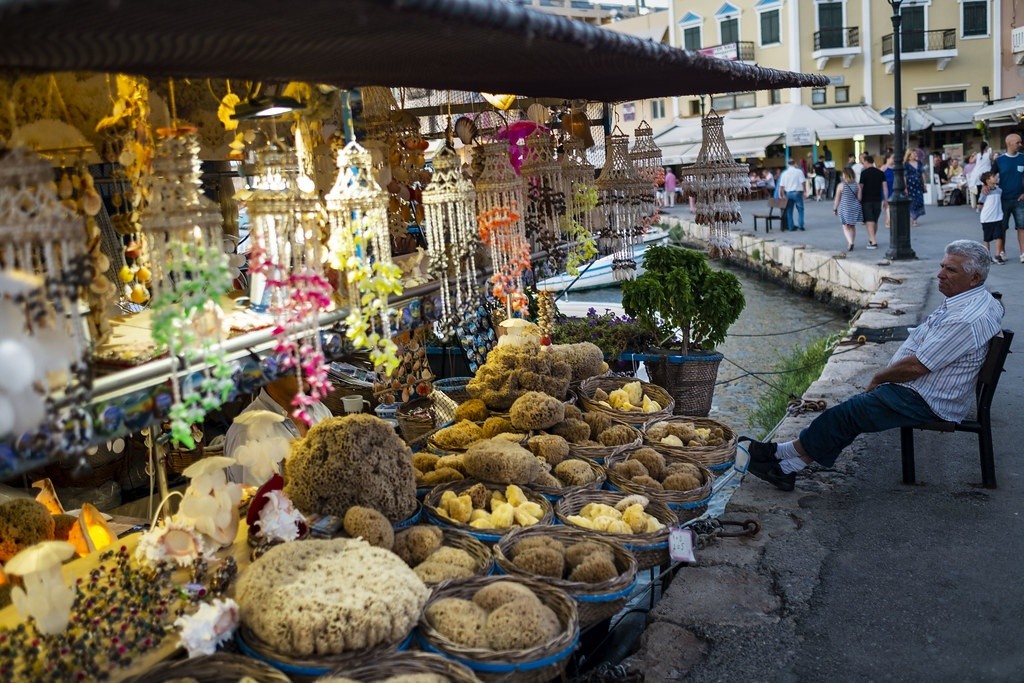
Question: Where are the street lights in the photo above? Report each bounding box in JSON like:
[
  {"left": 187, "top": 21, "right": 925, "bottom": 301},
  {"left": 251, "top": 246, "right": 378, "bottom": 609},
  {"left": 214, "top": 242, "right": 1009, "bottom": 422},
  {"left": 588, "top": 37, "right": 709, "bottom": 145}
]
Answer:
[{"left": 886, "top": 0, "right": 917, "bottom": 260}]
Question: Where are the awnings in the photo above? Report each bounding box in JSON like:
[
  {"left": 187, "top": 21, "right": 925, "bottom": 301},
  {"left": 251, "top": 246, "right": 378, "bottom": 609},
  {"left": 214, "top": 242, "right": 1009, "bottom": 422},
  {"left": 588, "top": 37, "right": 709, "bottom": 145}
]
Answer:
[
  {"left": 820, "top": 96, "right": 1024, "bottom": 140},
  {"left": 0, "top": 0, "right": 830, "bottom": 101},
  {"left": 588, "top": 102, "right": 822, "bottom": 169}
]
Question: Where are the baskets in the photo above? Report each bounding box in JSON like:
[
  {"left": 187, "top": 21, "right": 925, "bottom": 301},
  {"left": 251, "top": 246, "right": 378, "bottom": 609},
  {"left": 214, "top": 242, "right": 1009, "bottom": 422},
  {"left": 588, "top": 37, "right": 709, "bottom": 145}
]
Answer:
[
  {"left": 88, "top": 437, "right": 124, "bottom": 466},
  {"left": 166, "top": 435, "right": 227, "bottom": 474},
  {"left": 120, "top": 364, "right": 738, "bottom": 683}
]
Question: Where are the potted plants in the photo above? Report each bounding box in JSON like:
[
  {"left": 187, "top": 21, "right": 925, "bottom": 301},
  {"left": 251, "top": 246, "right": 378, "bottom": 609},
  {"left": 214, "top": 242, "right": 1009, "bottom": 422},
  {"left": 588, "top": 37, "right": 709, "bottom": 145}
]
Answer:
[{"left": 621, "top": 242, "right": 746, "bottom": 417}]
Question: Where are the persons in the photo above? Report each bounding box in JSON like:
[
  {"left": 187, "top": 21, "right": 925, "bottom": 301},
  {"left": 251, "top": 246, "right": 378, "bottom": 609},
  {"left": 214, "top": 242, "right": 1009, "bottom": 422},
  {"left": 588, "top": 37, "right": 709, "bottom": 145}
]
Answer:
[
  {"left": 904, "top": 147, "right": 926, "bottom": 226},
  {"left": 833, "top": 167, "right": 863, "bottom": 251},
  {"left": 650, "top": 145, "right": 896, "bottom": 233},
  {"left": 859, "top": 156, "right": 889, "bottom": 249},
  {"left": 977, "top": 171, "right": 1005, "bottom": 264},
  {"left": 736, "top": 240, "right": 1004, "bottom": 492},
  {"left": 930, "top": 141, "right": 1002, "bottom": 212},
  {"left": 981, "top": 133, "right": 1024, "bottom": 263}
]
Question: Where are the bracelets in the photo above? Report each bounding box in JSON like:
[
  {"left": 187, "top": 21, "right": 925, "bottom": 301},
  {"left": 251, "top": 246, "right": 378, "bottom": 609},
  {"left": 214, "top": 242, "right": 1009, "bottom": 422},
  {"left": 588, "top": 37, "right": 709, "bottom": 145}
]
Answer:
[{"left": 834, "top": 208, "right": 838, "bottom": 212}]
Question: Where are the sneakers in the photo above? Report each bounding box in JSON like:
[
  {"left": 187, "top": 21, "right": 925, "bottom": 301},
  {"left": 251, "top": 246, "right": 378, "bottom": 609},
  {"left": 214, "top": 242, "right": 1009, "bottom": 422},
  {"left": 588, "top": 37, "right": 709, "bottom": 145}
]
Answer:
[{"left": 739, "top": 436, "right": 797, "bottom": 491}]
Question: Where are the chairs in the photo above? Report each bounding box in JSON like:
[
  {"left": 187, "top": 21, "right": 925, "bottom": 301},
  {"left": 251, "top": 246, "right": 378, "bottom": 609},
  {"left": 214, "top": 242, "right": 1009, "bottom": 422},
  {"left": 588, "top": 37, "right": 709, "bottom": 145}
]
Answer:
[
  {"left": 751, "top": 198, "right": 789, "bottom": 234},
  {"left": 901, "top": 330, "right": 1014, "bottom": 488}
]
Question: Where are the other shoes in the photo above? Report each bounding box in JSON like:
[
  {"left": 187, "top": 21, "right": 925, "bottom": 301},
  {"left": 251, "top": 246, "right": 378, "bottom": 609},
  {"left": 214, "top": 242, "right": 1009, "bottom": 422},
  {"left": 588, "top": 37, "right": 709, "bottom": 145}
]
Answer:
[
  {"left": 989, "top": 256, "right": 994, "bottom": 264},
  {"left": 799, "top": 227, "right": 805, "bottom": 231},
  {"left": 817, "top": 196, "right": 822, "bottom": 202},
  {"left": 912, "top": 221, "right": 917, "bottom": 226},
  {"left": 866, "top": 240, "right": 877, "bottom": 249},
  {"left": 789, "top": 228, "right": 796, "bottom": 231},
  {"left": 885, "top": 223, "right": 890, "bottom": 228},
  {"left": 992, "top": 255, "right": 1005, "bottom": 265},
  {"left": 848, "top": 243, "right": 854, "bottom": 251},
  {"left": 1000, "top": 251, "right": 1008, "bottom": 261},
  {"left": 1020, "top": 255, "right": 1024, "bottom": 262}
]
